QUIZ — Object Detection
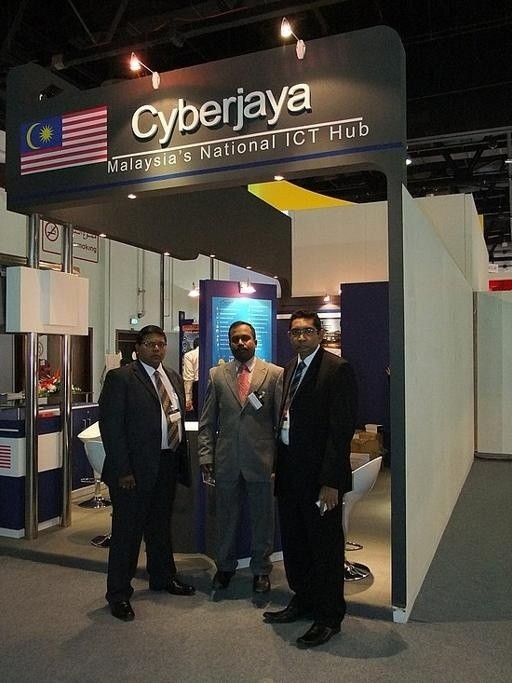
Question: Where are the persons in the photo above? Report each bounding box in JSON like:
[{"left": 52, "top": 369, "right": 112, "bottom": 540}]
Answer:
[
  {"left": 261, "top": 308, "right": 360, "bottom": 650},
  {"left": 181, "top": 336, "right": 199, "bottom": 420},
  {"left": 97, "top": 324, "right": 195, "bottom": 621},
  {"left": 196, "top": 321, "right": 286, "bottom": 602}
]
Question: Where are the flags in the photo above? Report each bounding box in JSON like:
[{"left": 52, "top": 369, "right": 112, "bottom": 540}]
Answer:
[{"left": 19, "top": 104, "right": 109, "bottom": 181}]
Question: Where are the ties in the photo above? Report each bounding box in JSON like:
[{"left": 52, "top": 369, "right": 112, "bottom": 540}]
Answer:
[
  {"left": 239, "top": 365, "right": 249, "bottom": 407},
  {"left": 154, "top": 371, "right": 180, "bottom": 453},
  {"left": 279, "top": 361, "right": 306, "bottom": 426}
]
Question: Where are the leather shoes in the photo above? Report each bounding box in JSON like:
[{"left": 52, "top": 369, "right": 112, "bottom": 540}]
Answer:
[
  {"left": 263, "top": 598, "right": 340, "bottom": 647},
  {"left": 110, "top": 601, "right": 134, "bottom": 621},
  {"left": 254, "top": 575, "right": 270, "bottom": 592},
  {"left": 212, "top": 570, "right": 229, "bottom": 589},
  {"left": 149, "top": 578, "right": 195, "bottom": 596}
]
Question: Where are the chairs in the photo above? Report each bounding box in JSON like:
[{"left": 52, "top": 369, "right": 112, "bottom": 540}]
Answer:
[
  {"left": 341, "top": 455, "right": 385, "bottom": 582},
  {"left": 77, "top": 421, "right": 111, "bottom": 508}
]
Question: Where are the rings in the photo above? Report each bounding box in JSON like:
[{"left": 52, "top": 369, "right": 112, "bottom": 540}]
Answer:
[{"left": 331, "top": 504, "right": 337, "bottom": 506}]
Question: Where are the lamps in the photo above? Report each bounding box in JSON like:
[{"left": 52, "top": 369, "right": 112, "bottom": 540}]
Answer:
[
  {"left": 129, "top": 51, "right": 160, "bottom": 90},
  {"left": 188, "top": 281, "right": 200, "bottom": 297},
  {"left": 241, "top": 277, "right": 256, "bottom": 293},
  {"left": 280, "top": 18, "right": 306, "bottom": 59}
]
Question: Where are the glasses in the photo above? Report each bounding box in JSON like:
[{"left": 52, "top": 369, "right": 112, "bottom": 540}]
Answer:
[{"left": 289, "top": 328, "right": 314, "bottom": 335}]
